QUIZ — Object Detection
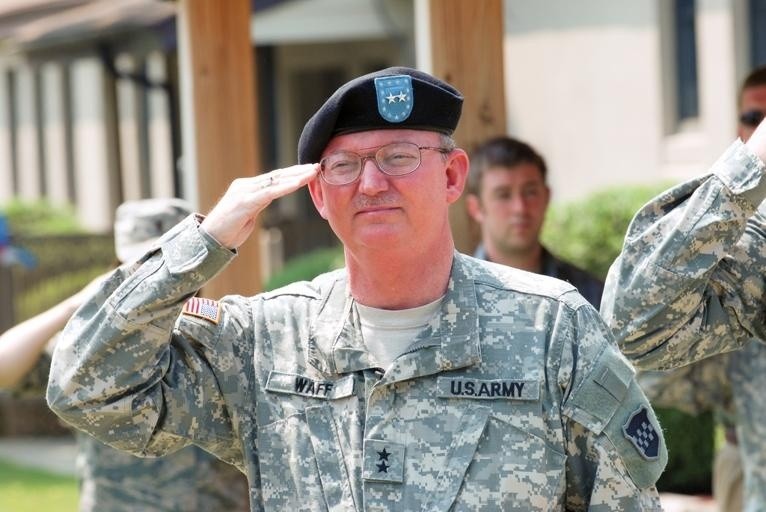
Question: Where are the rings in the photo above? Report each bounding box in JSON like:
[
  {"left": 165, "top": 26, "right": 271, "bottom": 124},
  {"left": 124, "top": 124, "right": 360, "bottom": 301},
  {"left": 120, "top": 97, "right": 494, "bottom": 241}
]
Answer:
[{"left": 260, "top": 175, "right": 274, "bottom": 189}]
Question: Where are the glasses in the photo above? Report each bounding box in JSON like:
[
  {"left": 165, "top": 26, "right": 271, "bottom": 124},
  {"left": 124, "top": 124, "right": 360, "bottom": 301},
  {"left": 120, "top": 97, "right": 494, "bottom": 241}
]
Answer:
[
  {"left": 319, "top": 143, "right": 449, "bottom": 185},
  {"left": 739, "top": 111, "right": 764, "bottom": 127}
]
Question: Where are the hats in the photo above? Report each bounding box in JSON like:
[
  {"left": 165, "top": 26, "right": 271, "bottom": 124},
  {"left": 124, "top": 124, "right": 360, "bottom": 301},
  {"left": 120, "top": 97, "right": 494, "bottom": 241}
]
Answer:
[
  {"left": 114, "top": 198, "right": 192, "bottom": 262},
  {"left": 298, "top": 67, "right": 463, "bottom": 165}
]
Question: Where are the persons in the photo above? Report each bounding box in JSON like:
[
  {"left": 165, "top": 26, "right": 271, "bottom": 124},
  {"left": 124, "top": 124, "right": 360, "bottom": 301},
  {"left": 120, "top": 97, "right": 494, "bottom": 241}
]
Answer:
[
  {"left": 594, "top": 114, "right": 765, "bottom": 512},
  {"left": 464, "top": 135, "right": 604, "bottom": 310},
  {"left": 0, "top": 196, "right": 250, "bottom": 512},
  {"left": 736, "top": 65, "right": 765, "bottom": 144},
  {"left": 44, "top": 62, "right": 669, "bottom": 512}
]
